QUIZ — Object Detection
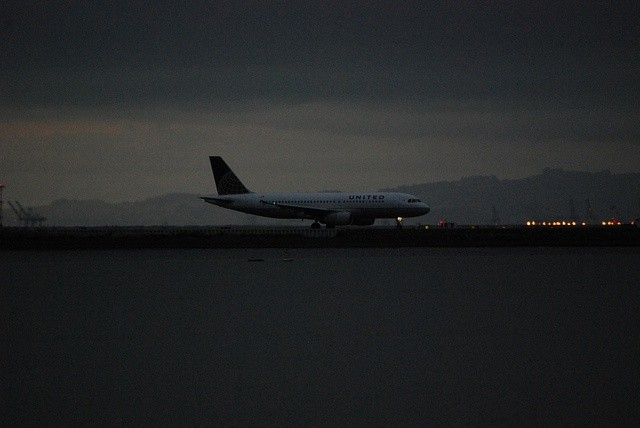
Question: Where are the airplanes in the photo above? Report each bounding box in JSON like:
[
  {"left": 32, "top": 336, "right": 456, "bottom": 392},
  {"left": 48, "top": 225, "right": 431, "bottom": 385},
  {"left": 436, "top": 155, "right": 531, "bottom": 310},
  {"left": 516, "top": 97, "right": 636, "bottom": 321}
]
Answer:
[
  {"left": 8, "top": 201, "right": 46, "bottom": 227},
  {"left": 200, "top": 155, "right": 432, "bottom": 238}
]
